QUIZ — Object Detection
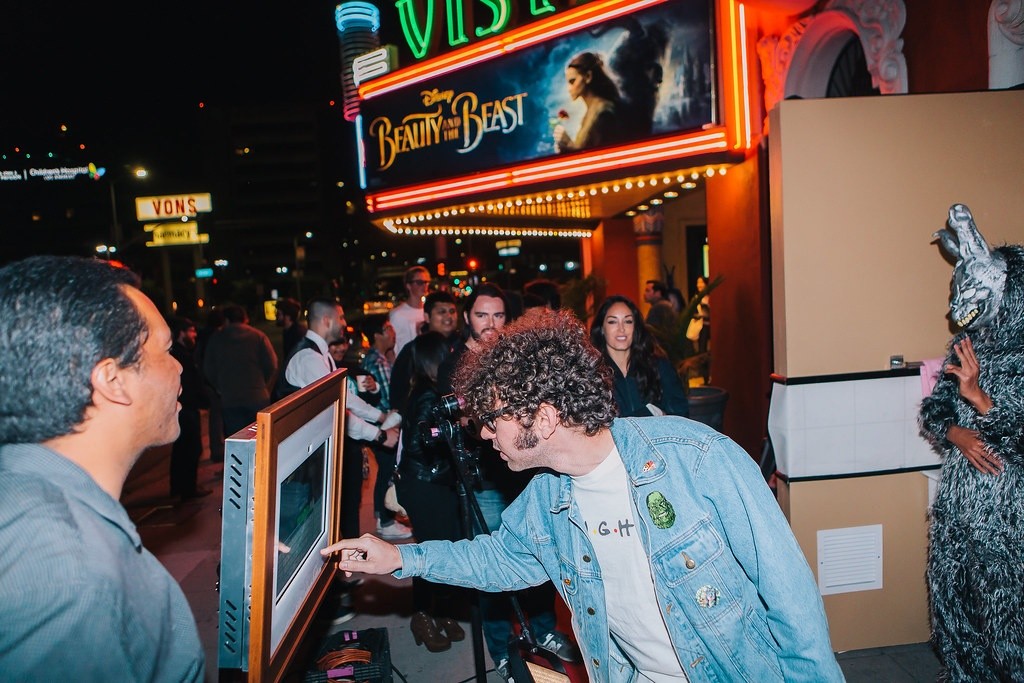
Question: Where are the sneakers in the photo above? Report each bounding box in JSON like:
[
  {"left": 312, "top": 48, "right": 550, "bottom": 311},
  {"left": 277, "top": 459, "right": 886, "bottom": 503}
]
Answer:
[
  {"left": 534, "top": 630, "right": 579, "bottom": 661},
  {"left": 495, "top": 657, "right": 516, "bottom": 683}
]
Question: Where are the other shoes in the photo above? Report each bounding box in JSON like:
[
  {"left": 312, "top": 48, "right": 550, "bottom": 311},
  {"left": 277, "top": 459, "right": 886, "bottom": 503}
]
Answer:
[
  {"left": 329, "top": 577, "right": 364, "bottom": 599},
  {"left": 180, "top": 486, "right": 214, "bottom": 501},
  {"left": 377, "top": 517, "right": 413, "bottom": 540},
  {"left": 314, "top": 600, "right": 360, "bottom": 625},
  {"left": 169, "top": 484, "right": 202, "bottom": 496},
  {"left": 211, "top": 443, "right": 224, "bottom": 463}
]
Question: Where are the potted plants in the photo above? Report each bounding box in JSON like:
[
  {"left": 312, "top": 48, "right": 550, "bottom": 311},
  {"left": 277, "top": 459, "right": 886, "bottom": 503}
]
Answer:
[{"left": 647, "top": 272, "right": 728, "bottom": 436}]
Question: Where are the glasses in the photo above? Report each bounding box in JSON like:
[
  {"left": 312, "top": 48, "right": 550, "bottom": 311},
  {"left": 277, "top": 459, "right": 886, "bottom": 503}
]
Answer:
[{"left": 409, "top": 280, "right": 431, "bottom": 286}]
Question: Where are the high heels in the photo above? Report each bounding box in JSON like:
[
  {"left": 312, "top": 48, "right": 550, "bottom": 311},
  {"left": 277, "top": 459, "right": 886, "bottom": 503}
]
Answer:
[
  {"left": 431, "top": 602, "right": 465, "bottom": 641},
  {"left": 410, "top": 610, "right": 451, "bottom": 652}
]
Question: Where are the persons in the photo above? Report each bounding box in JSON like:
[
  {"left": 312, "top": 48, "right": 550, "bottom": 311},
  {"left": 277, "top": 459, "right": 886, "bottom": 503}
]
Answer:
[
  {"left": 388, "top": 291, "right": 465, "bottom": 653},
  {"left": 504, "top": 280, "right": 561, "bottom": 325},
  {"left": 918, "top": 203, "right": 1024, "bottom": 683},
  {"left": 436, "top": 282, "right": 575, "bottom": 683},
  {"left": 589, "top": 295, "right": 688, "bottom": 418},
  {"left": 388, "top": 267, "right": 430, "bottom": 364},
  {"left": 169, "top": 318, "right": 214, "bottom": 500},
  {"left": 204, "top": 305, "right": 277, "bottom": 438},
  {"left": 320, "top": 306, "right": 846, "bottom": 683},
  {"left": 194, "top": 308, "right": 225, "bottom": 463},
  {"left": 275, "top": 300, "right": 307, "bottom": 357},
  {"left": 360, "top": 314, "right": 412, "bottom": 540},
  {"left": 0, "top": 255, "right": 207, "bottom": 683},
  {"left": 271, "top": 300, "right": 400, "bottom": 625},
  {"left": 643, "top": 280, "right": 684, "bottom": 364},
  {"left": 689, "top": 276, "right": 710, "bottom": 353}
]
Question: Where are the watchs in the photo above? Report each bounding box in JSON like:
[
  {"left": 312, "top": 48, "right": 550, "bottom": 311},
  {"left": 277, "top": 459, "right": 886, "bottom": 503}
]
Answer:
[{"left": 379, "top": 430, "right": 387, "bottom": 444}]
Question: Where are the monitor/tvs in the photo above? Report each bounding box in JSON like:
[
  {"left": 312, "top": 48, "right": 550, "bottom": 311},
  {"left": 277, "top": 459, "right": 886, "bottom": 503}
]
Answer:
[{"left": 215, "top": 366, "right": 348, "bottom": 683}]
